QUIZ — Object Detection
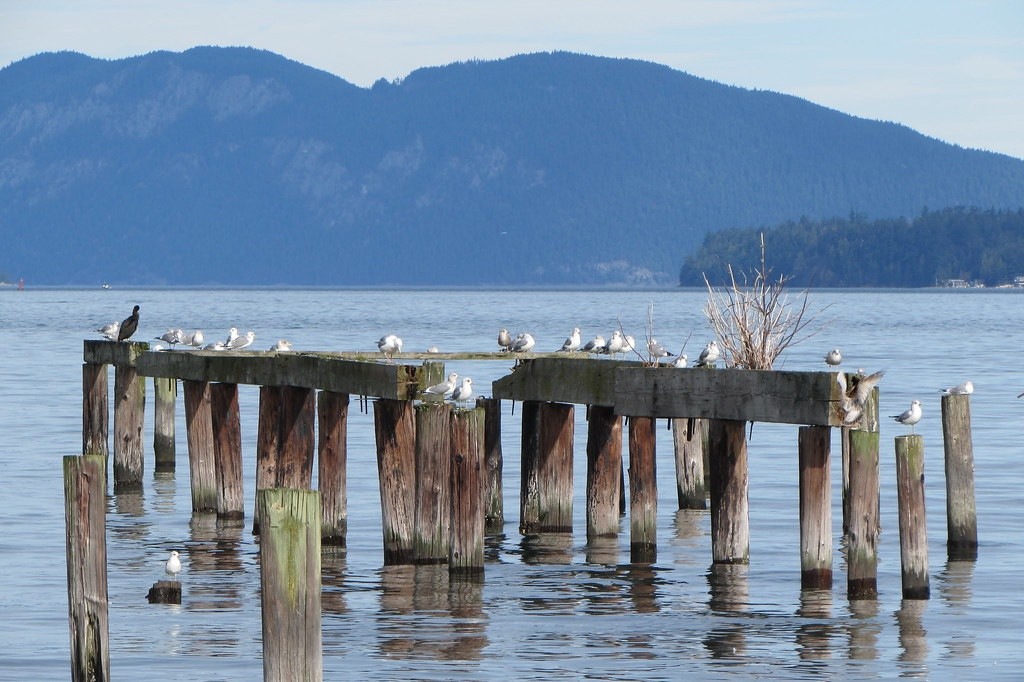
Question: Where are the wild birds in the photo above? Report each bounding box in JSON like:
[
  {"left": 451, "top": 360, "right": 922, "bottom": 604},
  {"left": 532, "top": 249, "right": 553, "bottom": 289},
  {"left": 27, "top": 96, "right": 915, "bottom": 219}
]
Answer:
[
  {"left": 91, "top": 321, "right": 120, "bottom": 341},
  {"left": 118, "top": 304, "right": 140, "bottom": 343},
  {"left": 427, "top": 344, "right": 440, "bottom": 353},
  {"left": 824, "top": 347, "right": 843, "bottom": 372},
  {"left": 645, "top": 332, "right": 673, "bottom": 370},
  {"left": 692, "top": 340, "right": 719, "bottom": 369},
  {"left": 154, "top": 326, "right": 255, "bottom": 351},
  {"left": 268, "top": 338, "right": 294, "bottom": 352},
  {"left": 555, "top": 325, "right": 635, "bottom": 359},
  {"left": 889, "top": 401, "right": 927, "bottom": 434},
  {"left": 937, "top": 380, "right": 975, "bottom": 395},
  {"left": 413, "top": 372, "right": 476, "bottom": 404},
  {"left": 667, "top": 353, "right": 690, "bottom": 370},
  {"left": 164, "top": 550, "right": 180, "bottom": 582},
  {"left": 374, "top": 333, "right": 402, "bottom": 364},
  {"left": 833, "top": 369, "right": 886, "bottom": 423},
  {"left": 495, "top": 327, "right": 535, "bottom": 352}
]
